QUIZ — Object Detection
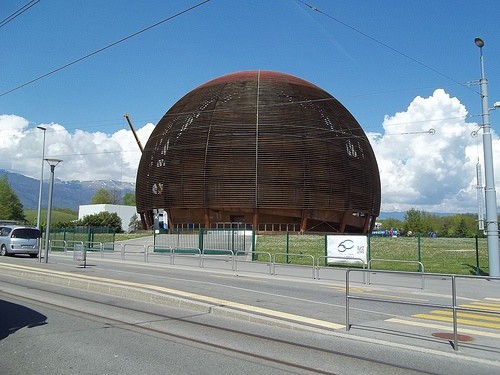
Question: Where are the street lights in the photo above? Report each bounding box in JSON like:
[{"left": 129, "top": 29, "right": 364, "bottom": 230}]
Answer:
[
  {"left": 475, "top": 38, "right": 500, "bottom": 278},
  {"left": 35, "top": 126, "right": 47, "bottom": 229}
]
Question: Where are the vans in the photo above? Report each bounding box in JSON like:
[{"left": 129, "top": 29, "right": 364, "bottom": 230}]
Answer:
[{"left": 0, "top": 225, "right": 41, "bottom": 256}]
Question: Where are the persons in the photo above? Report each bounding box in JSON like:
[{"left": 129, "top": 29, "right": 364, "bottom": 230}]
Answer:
[
  {"left": 384, "top": 227, "right": 389, "bottom": 237},
  {"left": 396, "top": 227, "right": 400, "bottom": 237},
  {"left": 390, "top": 227, "right": 394, "bottom": 237}
]
